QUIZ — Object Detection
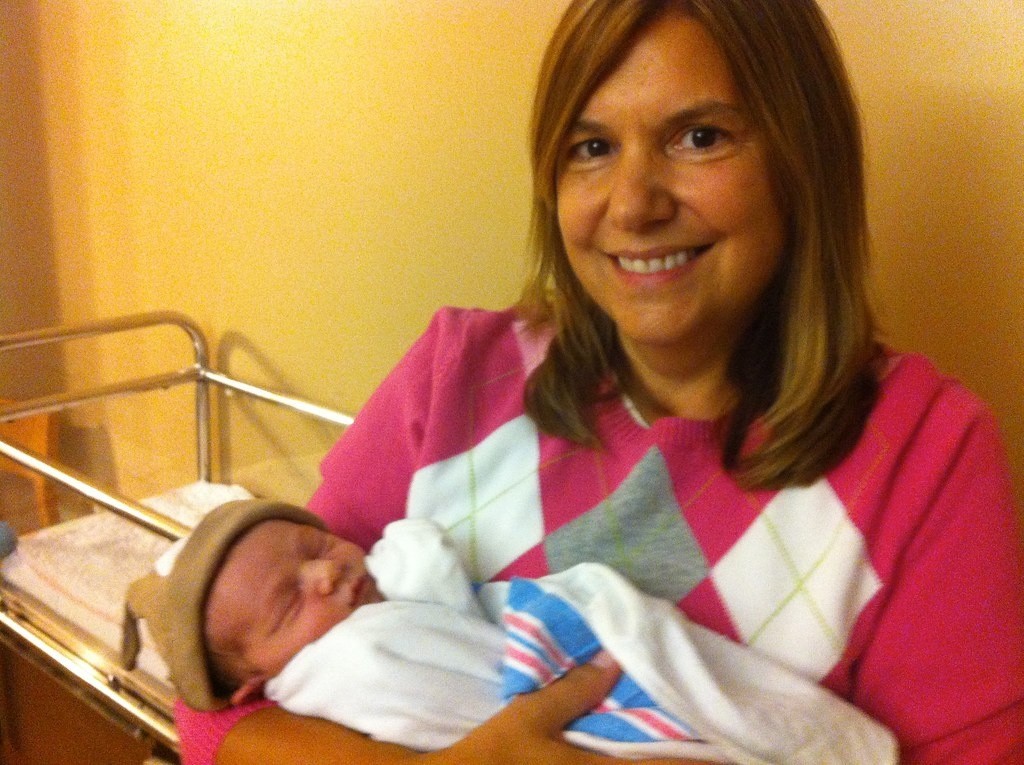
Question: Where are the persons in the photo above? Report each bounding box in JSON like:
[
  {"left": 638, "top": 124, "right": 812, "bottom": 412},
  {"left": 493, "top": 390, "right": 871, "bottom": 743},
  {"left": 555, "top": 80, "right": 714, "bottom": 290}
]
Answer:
[
  {"left": 116, "top": 496, "right": 912, "bottom": 765},
  {"left": 170, "top": 0, "right": 1024, "bottom": 765}
]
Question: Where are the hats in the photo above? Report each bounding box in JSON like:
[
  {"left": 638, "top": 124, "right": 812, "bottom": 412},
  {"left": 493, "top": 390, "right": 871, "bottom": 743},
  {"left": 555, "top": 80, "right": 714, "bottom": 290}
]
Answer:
[{"left": 120, "top": 496, "right": 330, "bottom": 718}]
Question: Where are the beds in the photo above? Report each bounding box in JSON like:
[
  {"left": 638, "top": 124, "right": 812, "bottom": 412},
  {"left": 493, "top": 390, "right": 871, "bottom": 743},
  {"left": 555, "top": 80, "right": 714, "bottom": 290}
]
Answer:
[{"left": 1, "top": 308, "right": 353, "bottom": 754}]
